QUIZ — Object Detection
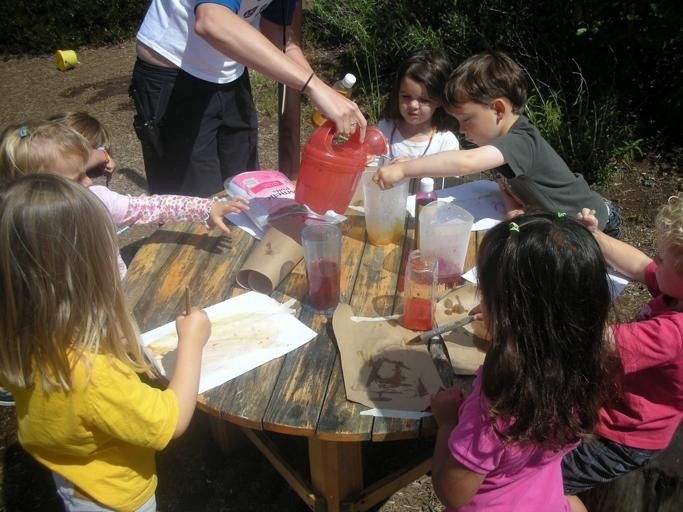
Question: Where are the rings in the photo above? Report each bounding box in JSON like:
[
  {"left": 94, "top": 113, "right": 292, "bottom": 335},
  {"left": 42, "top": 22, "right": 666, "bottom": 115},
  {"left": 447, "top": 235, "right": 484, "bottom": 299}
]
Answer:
[{"left": 351, "top": 121, "right": 357, "bottom": 128}]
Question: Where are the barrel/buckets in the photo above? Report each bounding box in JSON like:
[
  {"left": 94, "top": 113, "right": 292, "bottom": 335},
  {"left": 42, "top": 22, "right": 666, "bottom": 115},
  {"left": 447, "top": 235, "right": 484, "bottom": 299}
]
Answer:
[{"left": 56, "top": 49, "right": 78, "bottom": 71}]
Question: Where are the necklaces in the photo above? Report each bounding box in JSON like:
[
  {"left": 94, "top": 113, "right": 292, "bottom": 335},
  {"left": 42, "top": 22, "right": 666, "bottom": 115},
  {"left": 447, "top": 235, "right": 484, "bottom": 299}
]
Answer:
[{"left": 390, "top": 120, "right": 436, "bottom": 164}]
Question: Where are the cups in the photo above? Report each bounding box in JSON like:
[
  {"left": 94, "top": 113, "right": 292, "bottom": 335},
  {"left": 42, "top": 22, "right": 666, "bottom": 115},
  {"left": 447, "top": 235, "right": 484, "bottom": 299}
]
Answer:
[
  {"left": 418, "top": 201, "right": 472, "bottom": 279},
  {"left": 361, "top": 169, "right": 410, "bottom": 245},
  {"left": 302, "top": 224, "right": 342, "bottom": 309}
]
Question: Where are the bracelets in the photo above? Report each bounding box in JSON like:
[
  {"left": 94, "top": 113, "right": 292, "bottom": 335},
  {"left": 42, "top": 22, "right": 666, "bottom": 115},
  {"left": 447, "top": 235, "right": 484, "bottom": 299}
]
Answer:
[
  {"left": 102, "top": 150, "right": 109, "bottom": 163},
  {"left": 301, "top": 73, "right": 314, "bottom": 95}
]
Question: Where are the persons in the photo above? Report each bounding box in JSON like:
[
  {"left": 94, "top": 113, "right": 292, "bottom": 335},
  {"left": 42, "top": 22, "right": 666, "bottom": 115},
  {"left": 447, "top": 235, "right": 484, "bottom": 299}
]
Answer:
[
  {"left": 374, "top": 49, "right": 462, "bottom": 164},
  {"left": 561, "top": 194, "right": 683, "bottom": 512},
  {"left": 0, "top": 170, "right": 213, "bottom": 512},
  {"left": 48, "top": 111, "right": 117, "bottom": 187},
  {"left": 127, "top": 0, "right": 368, "bottom": 199},
  {"left": 430, "top": 211, "right": 632, "bottom": 511},
  {"left": 0, "top": 120, "right": 250, "bottom": 411},
  {"left": 370, "top": 49, "right": 622, "bottom": 239}
]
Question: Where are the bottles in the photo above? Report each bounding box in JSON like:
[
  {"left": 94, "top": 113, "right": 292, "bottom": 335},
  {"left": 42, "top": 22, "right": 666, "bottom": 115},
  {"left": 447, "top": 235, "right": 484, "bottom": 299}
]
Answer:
[
  {"left": 414, "top": 178, "right": 436, "bottom": 247},
  {"left": 402, "top": 246, "right": 439, "bottom": 331},
  {"left": 310, "top": 74, "right": 355, "bottom": 130}
]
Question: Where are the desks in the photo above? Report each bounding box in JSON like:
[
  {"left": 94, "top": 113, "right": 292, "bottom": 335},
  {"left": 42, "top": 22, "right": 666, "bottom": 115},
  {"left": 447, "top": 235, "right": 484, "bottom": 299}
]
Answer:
[{"left": 121, "top": 185, "right": 495, "bottom": 510}]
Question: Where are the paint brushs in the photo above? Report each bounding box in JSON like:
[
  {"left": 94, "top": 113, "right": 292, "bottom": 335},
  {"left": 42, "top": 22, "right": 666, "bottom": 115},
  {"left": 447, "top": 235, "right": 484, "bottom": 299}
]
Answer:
[
  {"left": 185, "top": 287, "right": 192, "bottom": 316},
  {"left": 404, "top": 313, "right": 476, "bottom": 346},
  {"left": 590, "top": 209, "right": 597, "bottom": 215}
]
